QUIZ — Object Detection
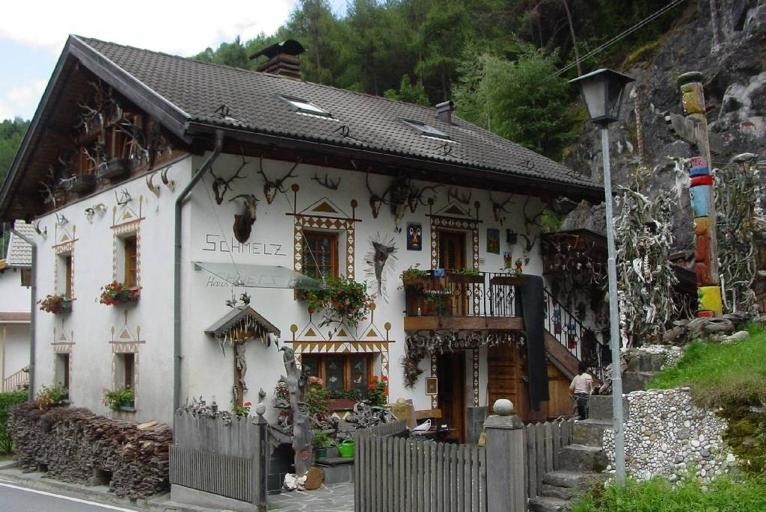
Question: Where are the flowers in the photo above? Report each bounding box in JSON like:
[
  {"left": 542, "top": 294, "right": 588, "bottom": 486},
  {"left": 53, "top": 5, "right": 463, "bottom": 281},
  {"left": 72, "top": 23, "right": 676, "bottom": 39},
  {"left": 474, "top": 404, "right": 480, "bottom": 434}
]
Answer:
[
  {"left": 304, "top": 273, "right": 376, "bottom": 319},
  {"left": 36, "top": 294, "right": 64, "bottom": 315},
  {"left": 307, "top": 375, "right": 390, "bottom": 415},
  {"left": 230, "top": 399, "right": 252, "bottom": 416},
  {"left": 99, "top": 280, "right": 129, "bottom": 306}
]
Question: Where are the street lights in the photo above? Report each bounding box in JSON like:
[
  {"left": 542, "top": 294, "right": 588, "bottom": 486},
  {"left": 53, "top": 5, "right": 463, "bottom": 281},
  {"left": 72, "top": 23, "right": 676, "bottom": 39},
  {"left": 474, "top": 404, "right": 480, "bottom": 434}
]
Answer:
[{"left": 569, "top": 68, "right": 636, "bottom": 511}]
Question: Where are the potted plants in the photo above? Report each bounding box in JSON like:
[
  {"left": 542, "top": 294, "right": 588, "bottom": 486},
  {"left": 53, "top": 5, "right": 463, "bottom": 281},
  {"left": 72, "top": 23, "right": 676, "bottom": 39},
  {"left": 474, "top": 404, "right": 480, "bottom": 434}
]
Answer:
[{"left": 99, "top": 384, "right": 134, "bottom": 413}]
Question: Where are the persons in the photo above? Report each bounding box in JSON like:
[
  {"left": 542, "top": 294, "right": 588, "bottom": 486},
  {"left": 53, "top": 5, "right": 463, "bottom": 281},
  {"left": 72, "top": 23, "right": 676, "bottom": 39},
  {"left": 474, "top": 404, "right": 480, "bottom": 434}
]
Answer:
[{"left": 568, "top": 360, "right": 594, "bottom": 421}]
